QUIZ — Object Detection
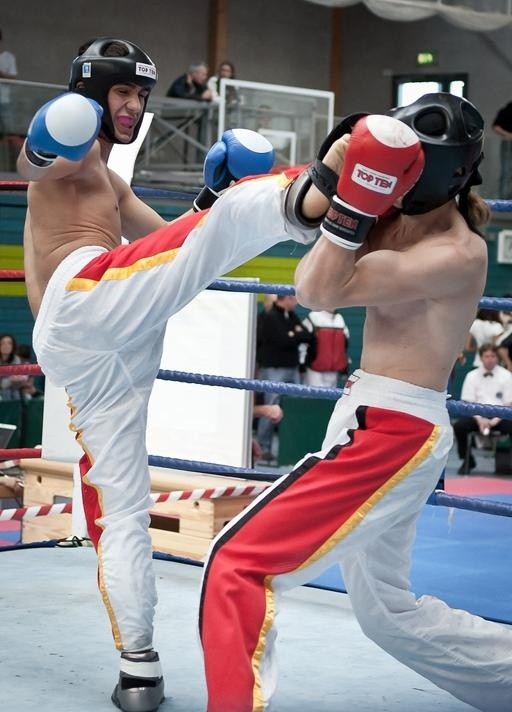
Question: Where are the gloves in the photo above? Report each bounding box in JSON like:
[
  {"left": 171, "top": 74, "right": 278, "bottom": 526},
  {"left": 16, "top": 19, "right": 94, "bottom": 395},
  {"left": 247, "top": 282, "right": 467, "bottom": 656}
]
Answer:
[
  {"left": 24, "top": 91, "right": 105, "bottom": 169},
  {"left": 194, "top": 129, "right": 275, "bottom": 213},
  {"left": 320, "top": 114, "right": 425, "bottom": 250}
]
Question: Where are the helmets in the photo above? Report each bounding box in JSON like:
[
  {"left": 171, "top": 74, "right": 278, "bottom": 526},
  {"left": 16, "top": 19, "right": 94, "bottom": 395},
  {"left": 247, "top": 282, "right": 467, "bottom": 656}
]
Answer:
[
  {"left": 385, "top": 93, "right": 485, "bottom": 216},
  {"left": 69, "top": 39, "right": 157, "bottom": 143}
]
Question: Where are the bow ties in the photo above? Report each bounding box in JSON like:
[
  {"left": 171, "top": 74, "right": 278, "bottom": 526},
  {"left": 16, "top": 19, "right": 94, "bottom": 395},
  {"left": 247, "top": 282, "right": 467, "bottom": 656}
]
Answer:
[{"left": 484, "top": 373, "right": 493, "bottom": 376}]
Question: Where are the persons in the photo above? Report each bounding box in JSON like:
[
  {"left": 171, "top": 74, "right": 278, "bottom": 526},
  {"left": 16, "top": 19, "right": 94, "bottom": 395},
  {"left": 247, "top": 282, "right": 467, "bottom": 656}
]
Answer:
[
  {"left": 196, "top": 62, "right": 245, "bottom": 166},
  {"left": 197, "top": 95, "right": 512, "bottom": 712},
  {"left": 446, "top": 306, "right": 512, "bottom": 477},
  {"left": 16, "top": 37, "right": 372, "bottom": 712},
  {"left": 494, "top": 94, "right": 512, "bottom": 198},
  {"left": 1, "top": 334, "right": 46, "bottom": 398},
  {"left": 153, "top": 63, "right": 212, "bottom": 164},
  {"left": 251, "top": 292, "right": 351, "bottom": 468}
]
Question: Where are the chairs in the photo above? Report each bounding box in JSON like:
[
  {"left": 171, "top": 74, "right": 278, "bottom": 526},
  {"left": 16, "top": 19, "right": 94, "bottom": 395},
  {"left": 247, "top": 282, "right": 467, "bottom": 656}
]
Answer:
[{"left": 463, "top": 429, "right": 508, "bottom": 476}]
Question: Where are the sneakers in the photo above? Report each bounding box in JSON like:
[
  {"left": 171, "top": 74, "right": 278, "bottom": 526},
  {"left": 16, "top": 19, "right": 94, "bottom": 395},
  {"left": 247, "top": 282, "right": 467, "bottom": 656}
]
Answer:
[
  {"left": 458, "top": 455, "right": 475, "bottom": 474},
  {"left": 256, "top": 453, "right": 278, "bottom": 467}
]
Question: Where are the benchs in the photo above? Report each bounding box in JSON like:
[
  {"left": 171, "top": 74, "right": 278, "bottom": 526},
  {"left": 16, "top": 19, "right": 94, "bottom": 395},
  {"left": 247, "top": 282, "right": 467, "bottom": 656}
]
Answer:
[{"left": 20, "top": 455, "right": 250, "bottom": 563}]
